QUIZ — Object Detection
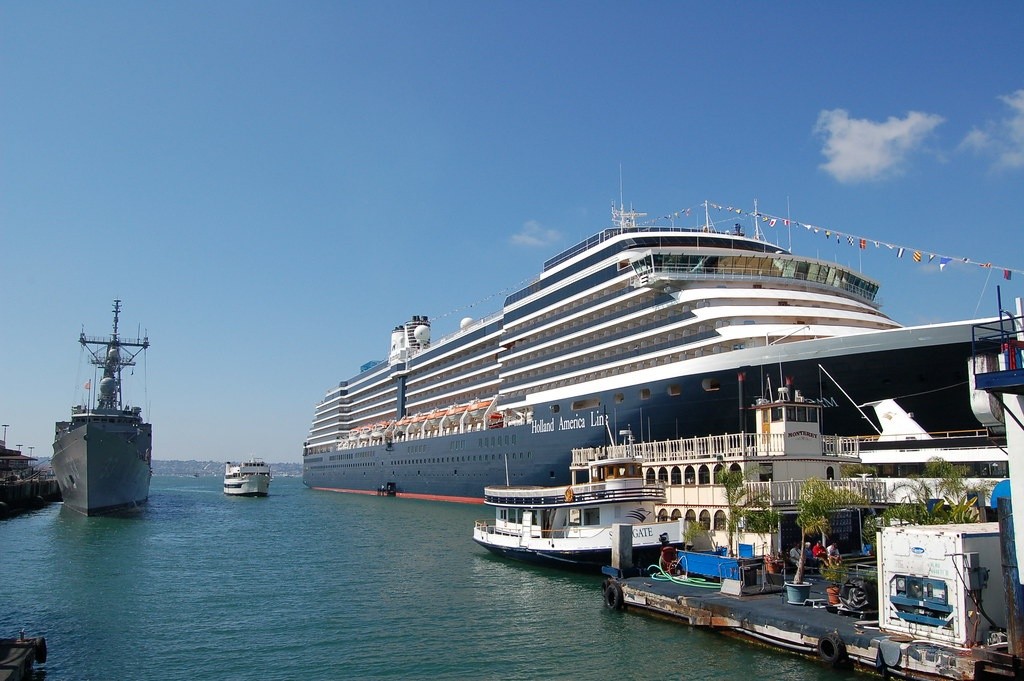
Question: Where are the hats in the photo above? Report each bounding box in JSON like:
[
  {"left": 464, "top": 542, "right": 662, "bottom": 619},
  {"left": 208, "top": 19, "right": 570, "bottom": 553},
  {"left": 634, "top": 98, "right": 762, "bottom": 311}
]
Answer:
[{"left": 817, "top": 540, "right": 821, "bottom": 544}]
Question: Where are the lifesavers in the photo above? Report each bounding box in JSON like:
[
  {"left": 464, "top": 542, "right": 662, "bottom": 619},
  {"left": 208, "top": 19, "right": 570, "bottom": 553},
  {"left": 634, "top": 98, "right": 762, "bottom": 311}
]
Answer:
[
  {"left": 816, "top": 634, "right": 846, "bottom": 663},
  {"left": 604, "top": 583, "right": 623, "bottom": 610},
  {"left": 564, "top": 489, "right": 574, "bottom": 503}
]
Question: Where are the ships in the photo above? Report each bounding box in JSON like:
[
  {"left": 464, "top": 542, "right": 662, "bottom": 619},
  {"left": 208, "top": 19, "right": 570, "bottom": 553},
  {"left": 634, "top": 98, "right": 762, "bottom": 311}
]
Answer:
[{"left": 303, "top": 162, "right": 1024, "bottom": 506}]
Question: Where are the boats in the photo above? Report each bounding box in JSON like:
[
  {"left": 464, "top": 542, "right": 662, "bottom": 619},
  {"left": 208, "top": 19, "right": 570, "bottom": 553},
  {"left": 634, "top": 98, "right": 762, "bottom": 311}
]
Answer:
[
  {"left": 0, "top": 631, "right": 48, "bottom": 680},
  {"left": 428, "top": 410, "right": 446, "bottom": 427},
  {"left": 52, "top": 295, "right": 153, "bottom": 519},
  {"left": 467, "top": 400, "right": 492, "bottom": 420},
  {"left": 470, "top": 427, "right": 687, "bottom": 570},
  {"left": 395, "top": 418, "right": 411, "bottom": 433},
  {"left": 446, "top": 405, "right": 468, "bottom": 423},
  {"left": 347, "top": 421, "right": 390, "bottom": 441},
  {"left": 222, "top": 456, "right": 271, "bottom": 497},
  {"left": 412, "top": 415, "right": 427, "bottom": 428}
]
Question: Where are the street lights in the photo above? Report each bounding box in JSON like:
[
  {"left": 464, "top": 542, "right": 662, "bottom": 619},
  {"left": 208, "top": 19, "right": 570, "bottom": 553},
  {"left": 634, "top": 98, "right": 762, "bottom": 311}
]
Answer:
[
  {"left": 28, "top": 447, "right": 35, "bottom": 457},
  {"left": 2, "top": 424, "right": 10, "bottom": 445},
  {"left": 16, "top": 445, "right": 23, "bottom": 451}
]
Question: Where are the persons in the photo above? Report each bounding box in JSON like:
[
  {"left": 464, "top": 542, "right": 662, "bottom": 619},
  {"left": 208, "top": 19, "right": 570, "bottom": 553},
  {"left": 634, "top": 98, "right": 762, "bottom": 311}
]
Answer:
[{"left": 790, "top": 540, "right": 842, "bottom": 581}]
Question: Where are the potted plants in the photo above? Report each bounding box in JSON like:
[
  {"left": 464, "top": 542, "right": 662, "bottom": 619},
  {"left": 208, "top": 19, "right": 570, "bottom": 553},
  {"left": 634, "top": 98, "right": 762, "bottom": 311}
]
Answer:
[{"left": 784, "top": 477, "right": 860, "bottom": 607}]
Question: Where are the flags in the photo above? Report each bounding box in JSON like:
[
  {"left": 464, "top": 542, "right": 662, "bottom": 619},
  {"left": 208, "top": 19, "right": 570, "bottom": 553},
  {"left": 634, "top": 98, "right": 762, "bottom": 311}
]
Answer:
[{"left": 636, "top": 203, "right": 1012, "bottom": 279}]
